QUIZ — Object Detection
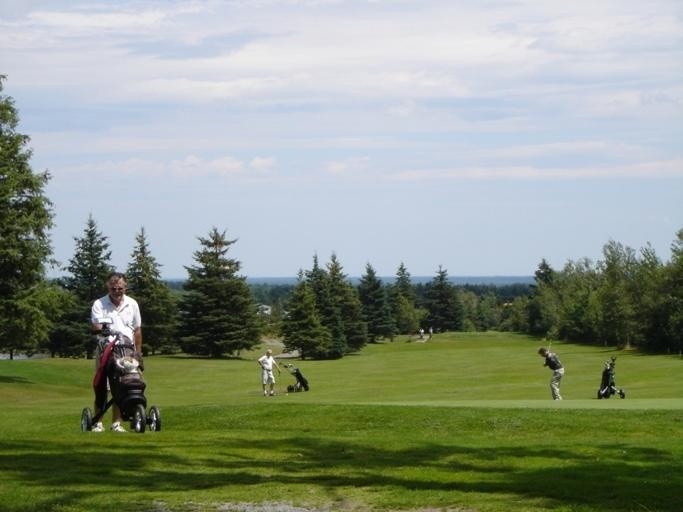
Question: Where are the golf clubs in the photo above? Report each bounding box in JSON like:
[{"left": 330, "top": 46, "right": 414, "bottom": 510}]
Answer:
[
  {"left": 280, "top": 363, "right": 296, "bottom": 373},
  {"left": 544, "top": 330, "right": 553, "bottom": 363},
  {"left": 95, "top": 327, "right": 126, "bottom": 357},
  {"left": 601, "top": 356, "right": 616, "bottom": 370}
]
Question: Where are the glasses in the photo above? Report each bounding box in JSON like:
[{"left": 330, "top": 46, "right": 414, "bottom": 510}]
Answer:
[{"left": 111, "top": 288, "right": 122, "bottom": 290}]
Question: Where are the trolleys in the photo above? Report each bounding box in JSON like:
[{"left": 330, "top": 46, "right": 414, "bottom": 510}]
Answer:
[
  {"left": 596, "top": 356, "right": 626, "bottom": 399},
  {"left": 277, "top": 361, "right": 309, "bottom": 392},
  {"left": 80, "top": 317, "right": 162, "bottom": 433}
]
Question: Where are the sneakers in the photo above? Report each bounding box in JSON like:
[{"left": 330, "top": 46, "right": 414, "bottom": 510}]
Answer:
[
  {"left": 264, "top": 393, "right": 276, "bottom": 396},
  {"left": 92, "top": 422, "right": 103, "bottom": 433},
  {"left": 112, "top": 422, "right": 126, "bottom": 432}
]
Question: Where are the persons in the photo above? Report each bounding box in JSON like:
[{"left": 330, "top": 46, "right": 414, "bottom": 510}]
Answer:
[
  {"left": 91, "top": 272, "right": 142, "bottom": 432},
  {"left": 419, "top": 327, "right": 433, "bottom": 339},
  {"left": 258, "top": 349, "right": 282, "bottom": 396},
  {"left": 538, "top": 346, "right": 565, "bottom": 401}
]
